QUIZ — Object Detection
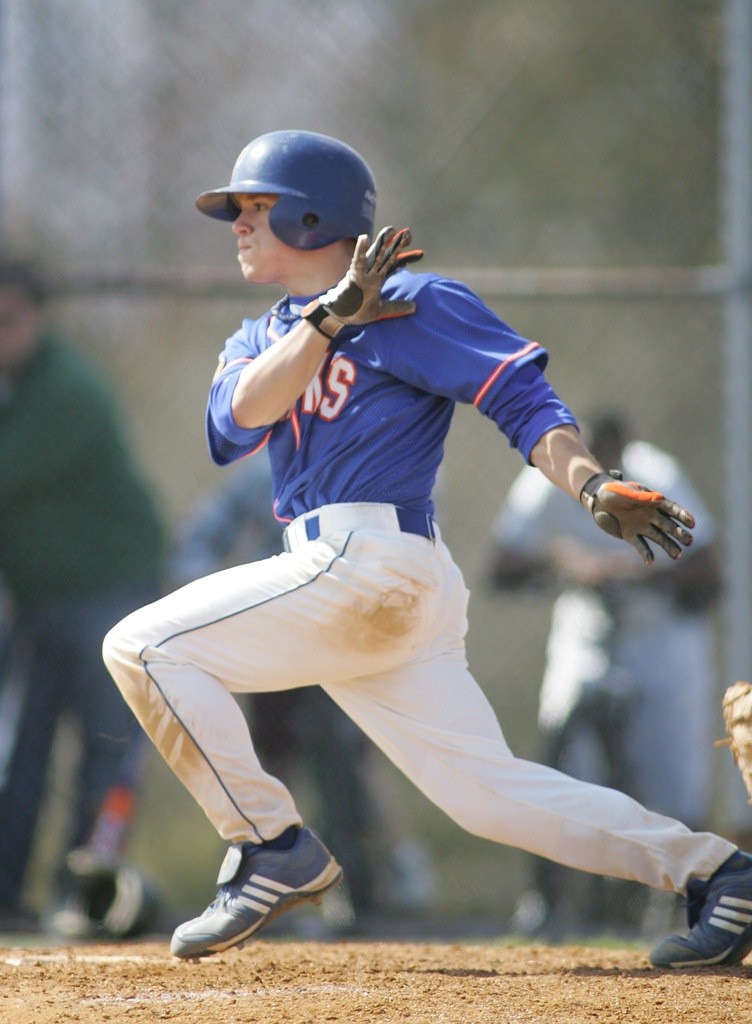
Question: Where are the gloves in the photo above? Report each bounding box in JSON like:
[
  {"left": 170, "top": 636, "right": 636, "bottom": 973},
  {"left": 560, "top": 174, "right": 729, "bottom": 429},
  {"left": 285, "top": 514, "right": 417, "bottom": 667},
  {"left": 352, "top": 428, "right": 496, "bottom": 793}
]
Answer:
[
  {"left": 579, "top": 470, "right": 695, "bottom": 565},
  {"left": 301, "top": 225, "right": 424, "bottom": 341}
]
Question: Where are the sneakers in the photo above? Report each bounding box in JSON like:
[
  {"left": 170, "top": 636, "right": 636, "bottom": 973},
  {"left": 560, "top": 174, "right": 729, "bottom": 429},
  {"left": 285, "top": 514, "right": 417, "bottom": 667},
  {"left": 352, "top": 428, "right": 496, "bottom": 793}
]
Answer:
[
  {"left": 649, "top": 862, "right": 752, "bottom": 969},
  {"left": 170, "top": 825, "right": 343, "bottom": 959}
]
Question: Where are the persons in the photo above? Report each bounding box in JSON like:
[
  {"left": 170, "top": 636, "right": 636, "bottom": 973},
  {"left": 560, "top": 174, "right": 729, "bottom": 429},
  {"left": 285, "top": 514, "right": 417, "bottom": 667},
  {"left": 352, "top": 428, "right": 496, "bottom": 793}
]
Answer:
[
  {"left": 102, "top": 129, "right": 752, "bottom": 970},
  {"left": 0, "top": 263, "right": 724, "bottom": 941}
]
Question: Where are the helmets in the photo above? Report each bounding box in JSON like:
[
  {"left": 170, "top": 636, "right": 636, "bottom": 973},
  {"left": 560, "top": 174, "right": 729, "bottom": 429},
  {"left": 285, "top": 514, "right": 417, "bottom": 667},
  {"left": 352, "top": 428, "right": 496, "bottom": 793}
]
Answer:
[{"left": 196, "top": 129, "right": 377, "bottom": 251}]
